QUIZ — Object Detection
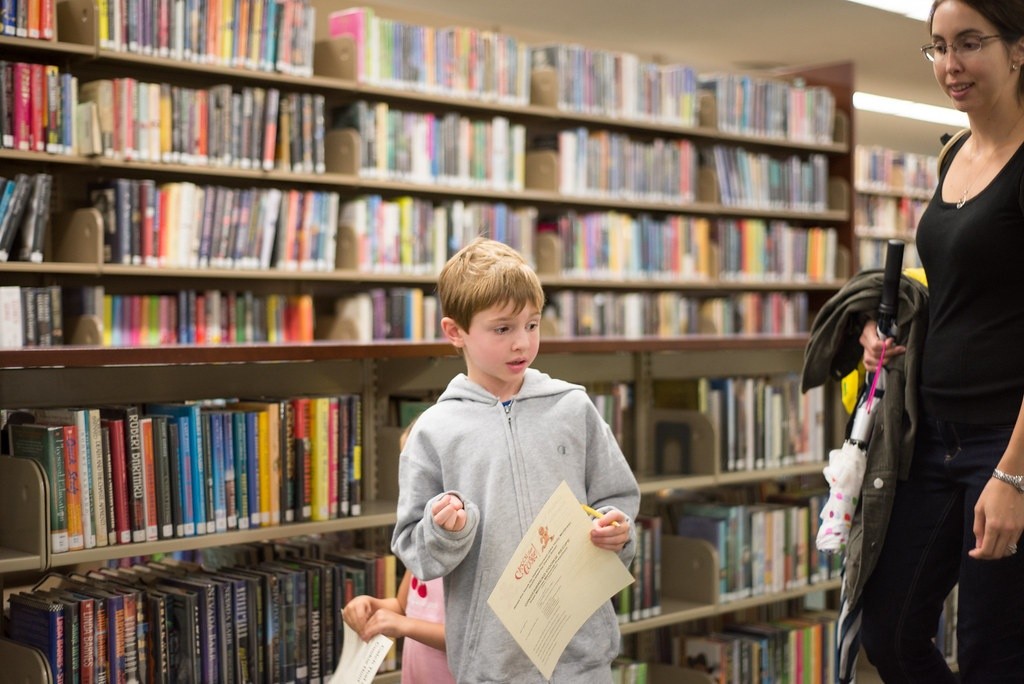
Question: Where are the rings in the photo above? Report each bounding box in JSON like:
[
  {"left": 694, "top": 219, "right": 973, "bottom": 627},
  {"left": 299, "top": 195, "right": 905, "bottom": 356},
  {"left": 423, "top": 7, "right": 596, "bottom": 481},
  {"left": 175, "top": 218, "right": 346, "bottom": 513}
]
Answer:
[{"left": 1007, "top": 543, "right": 1018, "bottom": 554}]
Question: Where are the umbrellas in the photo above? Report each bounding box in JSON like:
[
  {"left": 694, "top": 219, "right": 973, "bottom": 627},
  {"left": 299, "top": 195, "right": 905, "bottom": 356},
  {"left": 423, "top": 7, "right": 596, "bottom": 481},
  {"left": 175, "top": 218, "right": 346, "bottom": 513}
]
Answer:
[
  {"left": 831, "top": 238, "right": 909, "bottom": 684},
  {"left": 815, "top": 395, "right": 881, "bottom": 551}
]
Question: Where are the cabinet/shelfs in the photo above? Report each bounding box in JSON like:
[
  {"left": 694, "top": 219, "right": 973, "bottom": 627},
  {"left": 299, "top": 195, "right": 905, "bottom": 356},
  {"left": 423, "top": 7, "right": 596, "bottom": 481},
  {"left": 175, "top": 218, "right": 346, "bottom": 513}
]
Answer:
[{"left": 0, "top": 0, "right": 956, "bottom": 684}]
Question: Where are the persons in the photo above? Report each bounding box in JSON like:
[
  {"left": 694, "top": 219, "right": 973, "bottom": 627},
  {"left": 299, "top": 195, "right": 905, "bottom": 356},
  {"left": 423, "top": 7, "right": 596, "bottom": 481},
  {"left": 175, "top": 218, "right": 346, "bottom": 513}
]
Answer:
[
  {"left": 846, "top": 0, "right": 1024, "bottom": 684},
  {"left": 342, "top": 565, "right": 455, "bottom": 683},
  {"left": 389, "top": 234, "right": 643, "bottom": 684}
]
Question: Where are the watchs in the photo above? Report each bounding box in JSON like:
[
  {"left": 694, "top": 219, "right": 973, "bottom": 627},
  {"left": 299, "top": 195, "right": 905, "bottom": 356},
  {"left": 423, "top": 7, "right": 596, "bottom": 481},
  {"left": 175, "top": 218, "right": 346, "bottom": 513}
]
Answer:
[{"left": 988, "top": 468, "right": 1024, "bottom": 495}]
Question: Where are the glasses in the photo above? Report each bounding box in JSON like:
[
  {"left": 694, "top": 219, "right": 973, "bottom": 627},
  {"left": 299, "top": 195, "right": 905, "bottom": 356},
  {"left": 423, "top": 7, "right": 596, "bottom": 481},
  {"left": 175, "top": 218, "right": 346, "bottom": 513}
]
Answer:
[{"left": 920, "top": 33, "right": 1008, "bottom": 61}]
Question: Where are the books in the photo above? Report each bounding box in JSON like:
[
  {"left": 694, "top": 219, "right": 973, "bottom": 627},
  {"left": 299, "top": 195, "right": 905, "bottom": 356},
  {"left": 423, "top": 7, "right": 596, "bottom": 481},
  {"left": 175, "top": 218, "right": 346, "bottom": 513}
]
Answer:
[{"left": 4, "top": 1, "right": 968, "bottom": 684}]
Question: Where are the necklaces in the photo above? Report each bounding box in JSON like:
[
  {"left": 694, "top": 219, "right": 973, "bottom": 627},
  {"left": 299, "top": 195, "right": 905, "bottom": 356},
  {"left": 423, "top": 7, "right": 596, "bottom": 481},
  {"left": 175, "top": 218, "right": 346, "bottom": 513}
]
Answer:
[{"left": 955, "top": 103, "right": 1024, "bottom": 211}]
